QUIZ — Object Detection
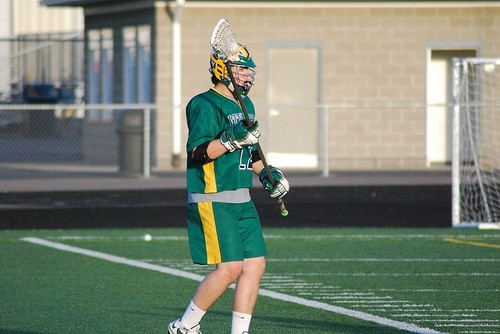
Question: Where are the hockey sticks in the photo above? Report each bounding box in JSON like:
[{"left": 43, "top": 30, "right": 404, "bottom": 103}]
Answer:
[{"left": 211, "top": 19, "right": 289, "bottom": 216}]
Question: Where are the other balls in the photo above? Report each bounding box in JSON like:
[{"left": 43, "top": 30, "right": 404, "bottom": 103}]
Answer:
[
  {"left": 144, "top": 234, "right": 151, "bottom": 241},
  {"left": 229, "top": 43, "right": 238, "bottom": 54}
]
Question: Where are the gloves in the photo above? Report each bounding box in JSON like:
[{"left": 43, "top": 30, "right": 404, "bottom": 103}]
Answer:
[
  {"left": 259, "top": 165, "right": 290, "bottom": 200},
  {"left": 220, "top": 119, "right": 261, "bottom": 152}
]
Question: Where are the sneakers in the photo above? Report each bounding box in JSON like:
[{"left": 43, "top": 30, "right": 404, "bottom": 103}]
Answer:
[
  {"left": 242, "top": 331, "right": 249, "bottom": 334},
  {"left": 168, "top": 318, "right": 203, "bottom": 334}
]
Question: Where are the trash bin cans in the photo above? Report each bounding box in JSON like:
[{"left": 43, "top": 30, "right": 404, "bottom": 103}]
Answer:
[{"left": 115, "top": 111, "right": 152, "bottom": 178}]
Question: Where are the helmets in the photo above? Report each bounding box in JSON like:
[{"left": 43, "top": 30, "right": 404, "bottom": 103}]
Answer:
[{"left": 208, "top": 18, "right": 256, "bottom": 96}]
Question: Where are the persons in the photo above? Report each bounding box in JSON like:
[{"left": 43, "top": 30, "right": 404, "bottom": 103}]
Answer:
[{"left": 167, "top": 18, "right": 291, "bottom": 334}]
